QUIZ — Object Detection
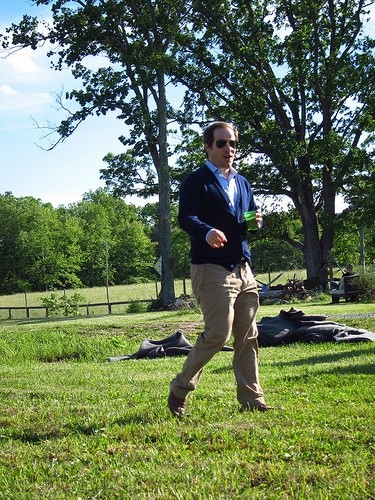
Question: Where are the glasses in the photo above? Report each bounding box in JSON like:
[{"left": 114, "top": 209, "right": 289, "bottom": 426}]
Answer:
[{"left": 215, "top": 139, "right": 237, "bottom": 149}]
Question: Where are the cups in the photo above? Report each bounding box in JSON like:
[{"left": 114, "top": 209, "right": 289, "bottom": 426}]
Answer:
[{"left": 244, "top": 211, "right": 259, "bottom": 231}]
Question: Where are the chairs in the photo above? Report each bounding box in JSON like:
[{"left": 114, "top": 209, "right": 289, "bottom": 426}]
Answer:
[{"left": 329, "top": 272, "right": 361, "bottom": 304}]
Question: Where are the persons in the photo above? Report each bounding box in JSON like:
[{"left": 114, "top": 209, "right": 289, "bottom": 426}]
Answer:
[
  {"left": 168, "top": 121, "right": 275, "bottom": 417},
  {"left": 331, "top": 264, "right": 358, "bottom": 290},
  {"left": 318, "top": 262, "right": 331, "bottom": 293}
]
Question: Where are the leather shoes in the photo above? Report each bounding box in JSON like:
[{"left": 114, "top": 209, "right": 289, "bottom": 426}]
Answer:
[
  {"left": 241, "top": 403, "right": 287, "bottom": 414},
  {"left": 167, "top": 378, "right": 187, "bottom": 419}
]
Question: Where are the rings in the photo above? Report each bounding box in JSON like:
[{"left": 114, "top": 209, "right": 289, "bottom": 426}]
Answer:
[{"left": 213, "top": 240, "right": 216, "bottom": 243}]
